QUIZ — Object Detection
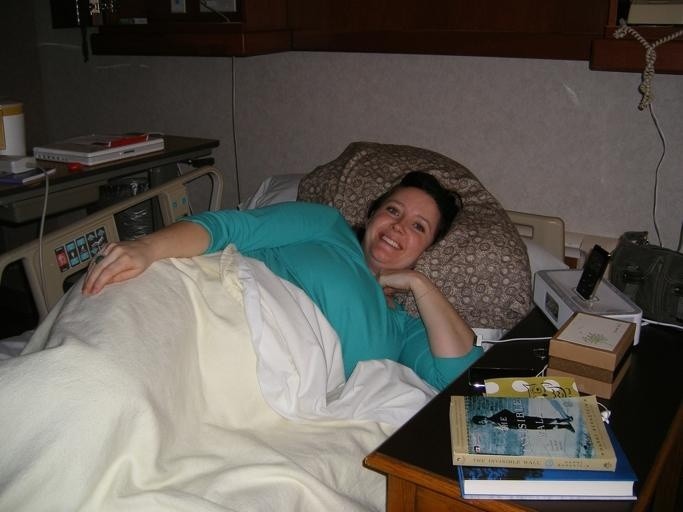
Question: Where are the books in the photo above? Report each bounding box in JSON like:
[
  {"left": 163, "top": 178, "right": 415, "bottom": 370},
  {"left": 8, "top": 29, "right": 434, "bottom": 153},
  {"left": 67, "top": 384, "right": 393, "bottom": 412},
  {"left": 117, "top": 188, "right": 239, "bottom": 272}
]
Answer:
[
  {"left": 449, "top": 393, "right": 617, "bottom": 472},
  {"left": 483, "top": 374, "right": 581, "bottom": 397},
  {"left": 457, "top": 420, "right": 638, "bottom": 500}
]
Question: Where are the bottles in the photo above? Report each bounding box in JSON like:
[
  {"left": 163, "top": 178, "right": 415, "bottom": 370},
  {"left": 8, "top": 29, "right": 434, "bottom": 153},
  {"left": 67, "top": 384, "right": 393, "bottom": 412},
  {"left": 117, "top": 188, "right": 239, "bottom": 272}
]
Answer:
[{"left": 0, "top": 99, "right": 27, "bottom": 158}]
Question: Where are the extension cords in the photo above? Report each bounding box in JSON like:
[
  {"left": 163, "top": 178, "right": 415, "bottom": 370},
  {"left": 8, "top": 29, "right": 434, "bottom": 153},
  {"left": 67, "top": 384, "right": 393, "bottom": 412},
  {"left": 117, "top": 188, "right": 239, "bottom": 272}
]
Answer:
[{"left": 0, "top": 152, "right": 34, "bottom": 173}]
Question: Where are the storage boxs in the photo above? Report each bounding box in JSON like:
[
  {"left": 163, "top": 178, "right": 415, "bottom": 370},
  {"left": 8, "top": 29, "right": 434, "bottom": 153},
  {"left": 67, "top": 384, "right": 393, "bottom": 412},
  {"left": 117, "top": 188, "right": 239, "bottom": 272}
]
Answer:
[{"left": 546, "top": 312, "right": 636, "bottom": 399}]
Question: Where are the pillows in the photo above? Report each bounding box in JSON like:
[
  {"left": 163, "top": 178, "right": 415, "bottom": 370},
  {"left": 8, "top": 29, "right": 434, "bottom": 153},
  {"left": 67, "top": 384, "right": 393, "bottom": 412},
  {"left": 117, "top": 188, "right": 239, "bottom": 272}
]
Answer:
[{"left": 299, "top": 141, "right": 538, "bottom": 322}]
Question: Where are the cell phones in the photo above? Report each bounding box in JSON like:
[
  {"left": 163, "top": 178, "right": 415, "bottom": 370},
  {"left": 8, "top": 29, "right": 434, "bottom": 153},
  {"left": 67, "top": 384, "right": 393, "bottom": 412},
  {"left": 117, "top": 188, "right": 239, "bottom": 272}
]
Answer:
[{"left": 578, "top": 244, "right": 610, "bottom": 302}]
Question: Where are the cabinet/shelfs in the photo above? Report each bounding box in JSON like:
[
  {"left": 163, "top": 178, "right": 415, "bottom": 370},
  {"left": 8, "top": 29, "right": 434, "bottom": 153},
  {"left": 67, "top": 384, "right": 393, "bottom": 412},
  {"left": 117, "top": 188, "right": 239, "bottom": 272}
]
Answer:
[
  {"left": 289, "top": 0, "right": 612, "bottom": 62},
  {"left": 48, "top": 1, "right": 295, "bottom": 58}
]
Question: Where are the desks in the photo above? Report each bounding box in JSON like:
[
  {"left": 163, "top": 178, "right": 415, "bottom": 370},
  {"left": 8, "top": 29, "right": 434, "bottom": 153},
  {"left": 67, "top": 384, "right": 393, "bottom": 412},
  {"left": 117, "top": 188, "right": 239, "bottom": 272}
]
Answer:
[
  {"left": 1, "top": 135, "right": 227, "bottom": 339},
  {"left": 357, "top": 307, "right": 682, "bottom": 512}
]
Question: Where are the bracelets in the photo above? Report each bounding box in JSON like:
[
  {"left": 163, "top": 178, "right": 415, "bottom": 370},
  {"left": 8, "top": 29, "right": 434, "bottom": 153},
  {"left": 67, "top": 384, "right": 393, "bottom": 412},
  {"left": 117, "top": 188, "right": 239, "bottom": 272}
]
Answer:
[{"left": 414, "top": 285, "right": 437, "bottom": 301}]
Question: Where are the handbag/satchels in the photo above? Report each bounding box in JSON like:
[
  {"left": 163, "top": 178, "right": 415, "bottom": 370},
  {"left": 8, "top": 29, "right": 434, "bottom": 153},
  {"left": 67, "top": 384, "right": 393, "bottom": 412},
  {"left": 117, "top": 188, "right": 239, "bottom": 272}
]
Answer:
[{"left": 610, "top": 231, "right": 683, "bottom": 326}]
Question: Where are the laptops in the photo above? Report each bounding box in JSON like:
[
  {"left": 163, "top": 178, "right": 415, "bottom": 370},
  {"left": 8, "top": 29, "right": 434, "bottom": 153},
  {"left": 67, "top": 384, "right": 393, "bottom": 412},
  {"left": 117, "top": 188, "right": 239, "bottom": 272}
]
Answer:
[{"left": 35, "top": 132, "right": 165, "bottom": 168}]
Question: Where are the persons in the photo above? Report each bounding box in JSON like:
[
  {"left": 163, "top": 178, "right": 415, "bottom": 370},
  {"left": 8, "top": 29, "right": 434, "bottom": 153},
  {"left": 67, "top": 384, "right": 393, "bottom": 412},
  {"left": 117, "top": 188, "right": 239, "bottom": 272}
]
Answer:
[
  {"left": 472, "top": 408, "right": 575, "bottom": 434},
  {"left": 81, "top": 170, "right": 485, "bottom": 393}
]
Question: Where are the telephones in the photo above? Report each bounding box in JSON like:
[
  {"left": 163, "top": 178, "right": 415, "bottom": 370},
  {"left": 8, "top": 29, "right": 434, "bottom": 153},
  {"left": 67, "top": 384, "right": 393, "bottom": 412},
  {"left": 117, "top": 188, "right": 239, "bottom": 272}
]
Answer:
[{"left": 532, "top": 244, "right": 644, "bottom": 347}]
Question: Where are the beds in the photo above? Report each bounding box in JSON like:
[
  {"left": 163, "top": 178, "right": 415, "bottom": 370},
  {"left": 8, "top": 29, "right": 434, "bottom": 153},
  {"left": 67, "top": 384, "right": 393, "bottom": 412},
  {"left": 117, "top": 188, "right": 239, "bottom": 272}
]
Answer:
[{"left": 4, "top": 173, "right": 565, "bottom": 512}]
